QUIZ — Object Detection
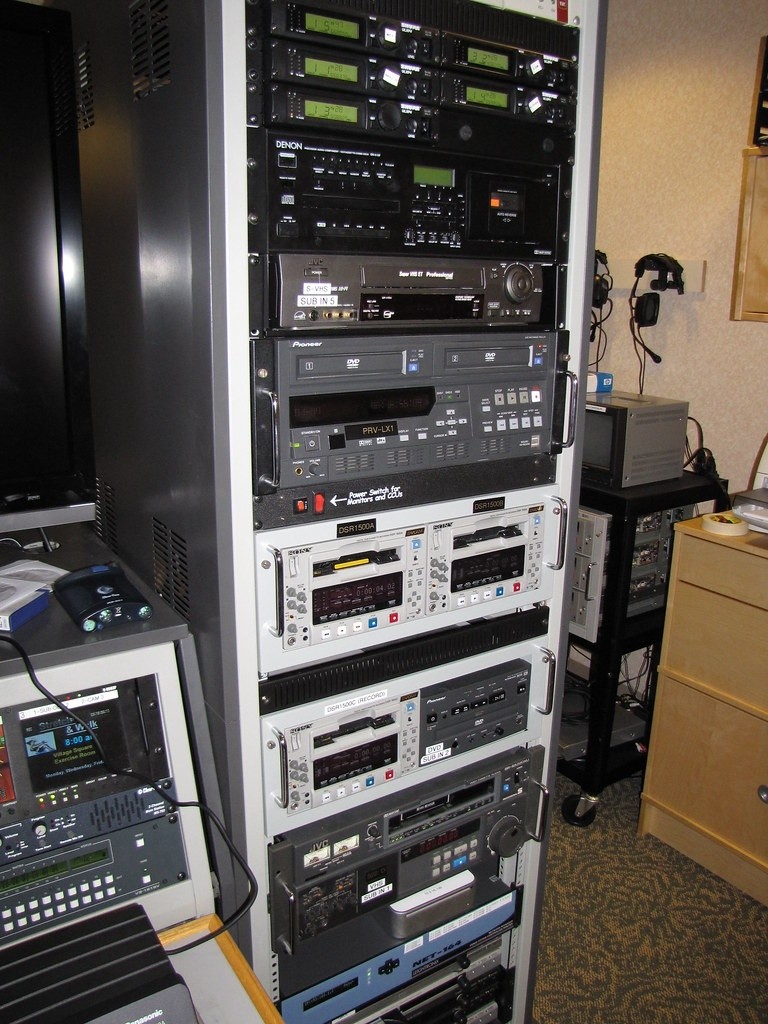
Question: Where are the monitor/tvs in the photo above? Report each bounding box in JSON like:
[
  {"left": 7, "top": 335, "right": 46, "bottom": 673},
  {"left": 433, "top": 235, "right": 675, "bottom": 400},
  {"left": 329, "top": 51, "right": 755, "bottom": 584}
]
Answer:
[
  {"left": 580, "top": 390, "right": 691, "bottom": 490},
  {"left": 0, "top": 679, "right": 154, "bottom": 819}
]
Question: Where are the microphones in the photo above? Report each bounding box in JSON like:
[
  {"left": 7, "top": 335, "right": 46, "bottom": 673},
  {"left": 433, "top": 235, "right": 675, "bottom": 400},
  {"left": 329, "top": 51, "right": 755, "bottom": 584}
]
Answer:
[
  {"left": 629, "top": 316, "right": 662, "bottom": 363},
  {"left": 589, "top": 309, "right": 597, "bottom": 342}
]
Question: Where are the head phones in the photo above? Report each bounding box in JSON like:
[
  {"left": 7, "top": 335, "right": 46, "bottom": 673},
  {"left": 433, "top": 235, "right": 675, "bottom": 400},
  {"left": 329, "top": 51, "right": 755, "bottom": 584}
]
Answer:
[
  {"left": 591, "top": 249, "right": 613, "bottom": 309},
  {"left": 629, "top": 252, "right": 685, "bottom": 327}
]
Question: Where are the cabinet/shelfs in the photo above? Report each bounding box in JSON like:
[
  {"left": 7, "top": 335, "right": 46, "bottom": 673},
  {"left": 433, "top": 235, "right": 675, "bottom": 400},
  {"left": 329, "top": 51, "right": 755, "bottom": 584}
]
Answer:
[
  {"left": 635, "top": 510, "right": 768, "bottom": 909},
  {"left": 556, "top": 471, "right": 729, "bottom": 828},
  {"left": 729, "top": 35, "right": 767, "bottom": 322}
]
